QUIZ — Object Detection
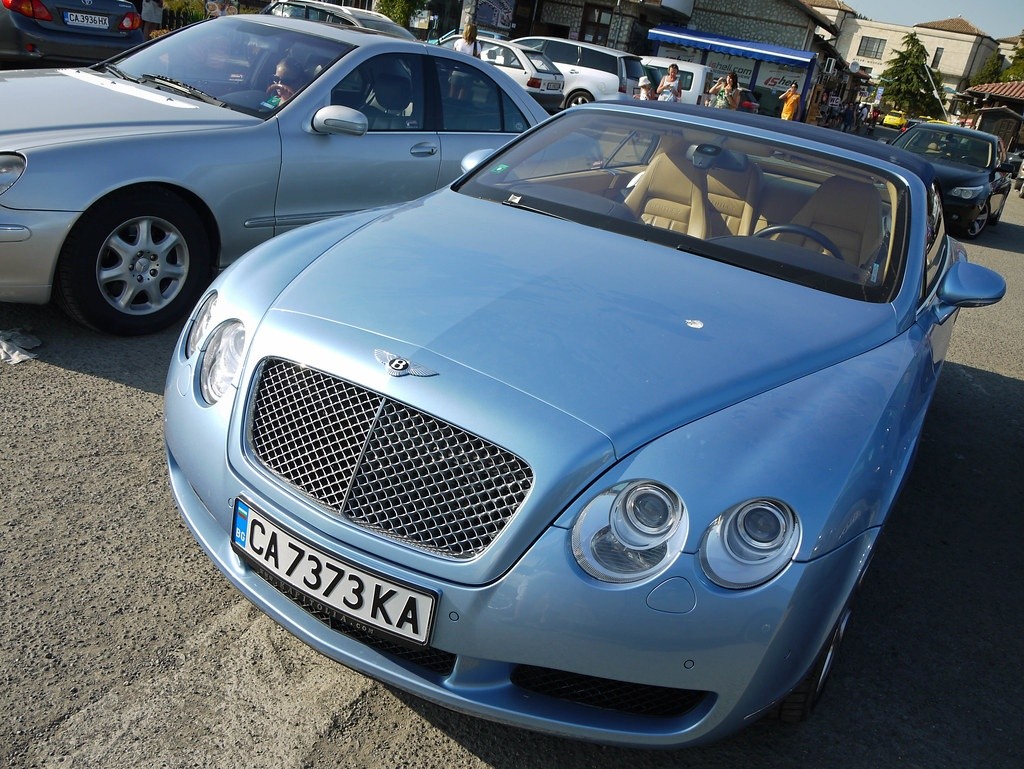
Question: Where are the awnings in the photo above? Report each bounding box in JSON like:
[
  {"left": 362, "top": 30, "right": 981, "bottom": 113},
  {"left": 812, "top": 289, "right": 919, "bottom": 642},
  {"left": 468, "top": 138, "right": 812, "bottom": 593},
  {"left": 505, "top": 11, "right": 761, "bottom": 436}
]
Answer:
[{"left": 648, "top": 24, "right": 817, "bottom": 69}]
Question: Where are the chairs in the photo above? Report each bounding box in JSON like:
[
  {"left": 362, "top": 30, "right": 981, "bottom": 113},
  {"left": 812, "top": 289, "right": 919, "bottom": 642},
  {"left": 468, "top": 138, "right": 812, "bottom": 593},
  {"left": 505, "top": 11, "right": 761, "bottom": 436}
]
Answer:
[
  {"left": 772, "top": 176, "right": 885, "bottom": 263},
  {"left": 303, "top": 55, "right": 349, "bottom": 95},
  {"left": 624, "top": 150, "right": 763, "bottom": 239},
  {"left": 355, "top": 75, "right": 420, "bottom": 130},
  {"left": 909, "top": 137, "right": 941, "bottom": 153},
  {"left": 484, "top": 50, "right": 496, "bottom": 63}
]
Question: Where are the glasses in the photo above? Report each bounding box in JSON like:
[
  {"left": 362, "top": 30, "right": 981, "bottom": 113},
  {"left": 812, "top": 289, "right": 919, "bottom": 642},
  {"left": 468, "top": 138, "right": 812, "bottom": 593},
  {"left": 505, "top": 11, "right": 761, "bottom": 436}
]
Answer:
[{"left": 271, "top": 73, "right": 299, "bottom": 85}]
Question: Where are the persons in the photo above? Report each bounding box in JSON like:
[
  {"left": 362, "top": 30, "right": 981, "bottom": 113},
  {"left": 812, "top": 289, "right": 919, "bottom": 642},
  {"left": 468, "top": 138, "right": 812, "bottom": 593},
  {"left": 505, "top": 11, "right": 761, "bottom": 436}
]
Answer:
[
  {"left": 141, "top": 0, "right": 164, "bottom": 42},
  {"left": 632, "top": 63, "right": 741, "bottom": 110},
  {"left": 259, "top": 57, "right": 305, "bottom": 109},
  {"left": 447, "top": 23, "right": 481, "bottom": 103},
  {"left": 779, "top": 83, "right": 801, "bottom": 121},
  {"left": 821, "top": 102, "right": 879, "bottom": 137}
]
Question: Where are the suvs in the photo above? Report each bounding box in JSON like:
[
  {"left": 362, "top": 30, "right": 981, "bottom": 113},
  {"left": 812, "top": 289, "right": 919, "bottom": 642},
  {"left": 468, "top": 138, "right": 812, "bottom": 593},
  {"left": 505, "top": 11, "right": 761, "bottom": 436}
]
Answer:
[
  {"left": 712, "top": 80, "right": 759, "bottom": 114},
  {"left": 248, "top": 0, "right": 415, "bottom": 80},
  {"left": 476, "top": 35, "right": 649, "bottom": 108}
]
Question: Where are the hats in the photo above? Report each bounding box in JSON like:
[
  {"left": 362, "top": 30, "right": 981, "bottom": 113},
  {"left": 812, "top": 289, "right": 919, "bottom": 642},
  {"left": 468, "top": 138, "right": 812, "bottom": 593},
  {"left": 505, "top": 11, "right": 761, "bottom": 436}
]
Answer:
[{"left": 638, "top": 75, "right": 651, "bottom": 85}]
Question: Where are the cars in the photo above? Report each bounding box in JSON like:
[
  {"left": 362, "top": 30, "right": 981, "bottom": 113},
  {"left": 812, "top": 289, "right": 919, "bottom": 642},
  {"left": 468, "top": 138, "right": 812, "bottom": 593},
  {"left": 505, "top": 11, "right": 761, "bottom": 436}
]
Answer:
[
  {"left": 1, "top": 15, "right": 550, "bottom": 338},
  {"left": 1005, "top": 148, "right": 1024, "bottom": 194},
  {"left": 0, "top": 0, "right": 147, "bottom": 72},
  {"left": 883, "top": 110, "right": 908, "bottom": 128},
  {"left": 888, "top": 121, "right": 1012, "bottom": 238},
  {"left": 856, "top": 101, "right": 883, "bottom": 125},
  {"left": 163, "top": 106, "right": 1005, "bottom": 761},
  {"left": 421, "top": 28, "right": 513, "bottom": 48},
  {"left": 430, "top": 34, "right": 563, "bottom": 111}
]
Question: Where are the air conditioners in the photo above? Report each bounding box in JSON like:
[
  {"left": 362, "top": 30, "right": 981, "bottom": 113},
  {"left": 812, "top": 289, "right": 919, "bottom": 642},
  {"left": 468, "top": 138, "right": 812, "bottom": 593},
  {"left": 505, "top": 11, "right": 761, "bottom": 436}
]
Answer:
[{"left": 823, "top": 57, "right": 836, "bottom": 76}]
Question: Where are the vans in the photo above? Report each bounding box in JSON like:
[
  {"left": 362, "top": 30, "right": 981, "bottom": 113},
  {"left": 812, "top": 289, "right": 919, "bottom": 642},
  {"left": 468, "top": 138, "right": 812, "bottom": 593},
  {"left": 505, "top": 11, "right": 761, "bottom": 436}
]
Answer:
[{"left": 640, "top": 57, "right": 714, "bottom": 105}]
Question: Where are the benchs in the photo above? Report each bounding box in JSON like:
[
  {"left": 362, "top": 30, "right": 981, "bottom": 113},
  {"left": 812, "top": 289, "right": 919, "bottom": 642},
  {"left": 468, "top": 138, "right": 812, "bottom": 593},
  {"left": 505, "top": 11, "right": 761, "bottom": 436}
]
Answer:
[{"left": 655, "top": 136, "right": 892, "bottom": 243}]
